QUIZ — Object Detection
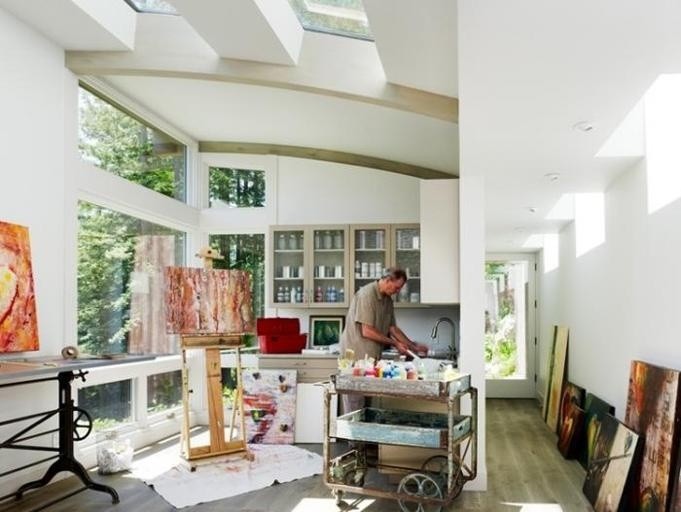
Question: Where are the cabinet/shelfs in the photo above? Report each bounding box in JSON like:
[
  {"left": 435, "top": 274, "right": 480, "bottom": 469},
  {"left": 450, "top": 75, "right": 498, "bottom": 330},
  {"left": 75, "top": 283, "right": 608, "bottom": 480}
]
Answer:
[
  {"left": 421, "top": 180, "right": 460, "bottom": 304},
  {"left": 351, "top": 223, "right": 430, "bottom": 307},
  {"left": 268, "top": 223, "right": 349, "bottom": 308},
  {"left": 260, "top": 359, "right": 338, "bottom": 384}
]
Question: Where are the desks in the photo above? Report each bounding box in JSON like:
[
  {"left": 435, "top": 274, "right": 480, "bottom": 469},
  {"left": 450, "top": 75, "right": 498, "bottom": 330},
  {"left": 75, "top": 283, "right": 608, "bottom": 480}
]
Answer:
[{"left": 0, "top": 356, "right": 156, "bottom": 512}]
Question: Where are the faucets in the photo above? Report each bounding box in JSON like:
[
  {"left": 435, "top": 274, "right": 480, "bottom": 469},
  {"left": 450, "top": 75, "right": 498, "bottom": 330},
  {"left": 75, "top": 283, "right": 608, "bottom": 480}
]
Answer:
[{"left": 431, "top": 317, "right": 457, "bottom": 355}]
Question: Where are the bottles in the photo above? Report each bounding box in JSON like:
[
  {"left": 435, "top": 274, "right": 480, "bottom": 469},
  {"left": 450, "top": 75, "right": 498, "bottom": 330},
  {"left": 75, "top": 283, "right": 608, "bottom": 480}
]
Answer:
[
  {"left": 323, "top": 231, "right": 332, "bottom": 248},
  {"left": 331, "top": 287, "right": 337, "bottom": 302},
  {"left": 375, "top": 263, "right": 383, "bottom": 278},
  {"left": 277, "top": 287, "right": 284, "bottom": 303},
  {"left": 412, "top": 235, "right": 419, "bottom": 248},
  {"left": 355, "top": 260, "right": 361, "bottom": 278},
  {"left": 338, "top": 287, "right": 344, "bottom": 302},
  {"left": 278, "top": 234, "right": 286, "bottom": 249},
  {"left": 326, "top": 287, "right": 331, "bottom": 302},
  {"left": 299, "top": 233, "right": 304, "bottom": 250},
  {"left": 290, "top": 287, "right": 296, "bottom": 303},
  {"left": 315, "top": 286, "right": 322, "bottom": 302},
  {"left": 314, "top": 231, "right": 322, "bottom": 249},
  {"left": 288, "top": 233, "right": 297, "bottom": 249},
  {"left": 398, "top": 282, "right": 409, "bottom": 302},
  {"left": 361, "top": 262, "right": 368, "bottom": 278},
  {"left": 410, "top": 292, "right": 419, "bottom": 302},
  {"left": 284, "top": 287, "right": 290, "bottom": 303},
  {"left": 370, "top": 262, "right": 376, "bottom": 278},
  {"left": 382, "top": 362, "right": 418, "bottom": 380},
  {"left": 297, "top": 286, "right": 302, "bottom": 303},
  {"left": 334, "top": 231, "right": 342, "bottom": 249}
]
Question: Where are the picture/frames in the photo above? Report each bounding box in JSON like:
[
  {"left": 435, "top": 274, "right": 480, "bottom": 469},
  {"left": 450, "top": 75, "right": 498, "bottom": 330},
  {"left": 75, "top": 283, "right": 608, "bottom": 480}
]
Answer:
[
  {"left": 240, "top": 369, "right": 296, "bottom": 444},
  {"left": 309, "top": 315, "right": 346, "bottom": 351}
]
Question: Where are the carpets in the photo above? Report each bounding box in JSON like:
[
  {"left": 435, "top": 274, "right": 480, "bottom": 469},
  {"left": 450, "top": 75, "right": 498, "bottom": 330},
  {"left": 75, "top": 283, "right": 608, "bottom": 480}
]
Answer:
[{"left": 134, "top": 443, "right": 324, "bottom": 507}]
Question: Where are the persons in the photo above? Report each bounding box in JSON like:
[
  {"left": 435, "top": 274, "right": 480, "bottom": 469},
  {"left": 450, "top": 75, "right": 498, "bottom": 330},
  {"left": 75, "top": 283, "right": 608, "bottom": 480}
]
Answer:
[{"left": 337, "top": 267, "right": 428, "bottom": 452}]
